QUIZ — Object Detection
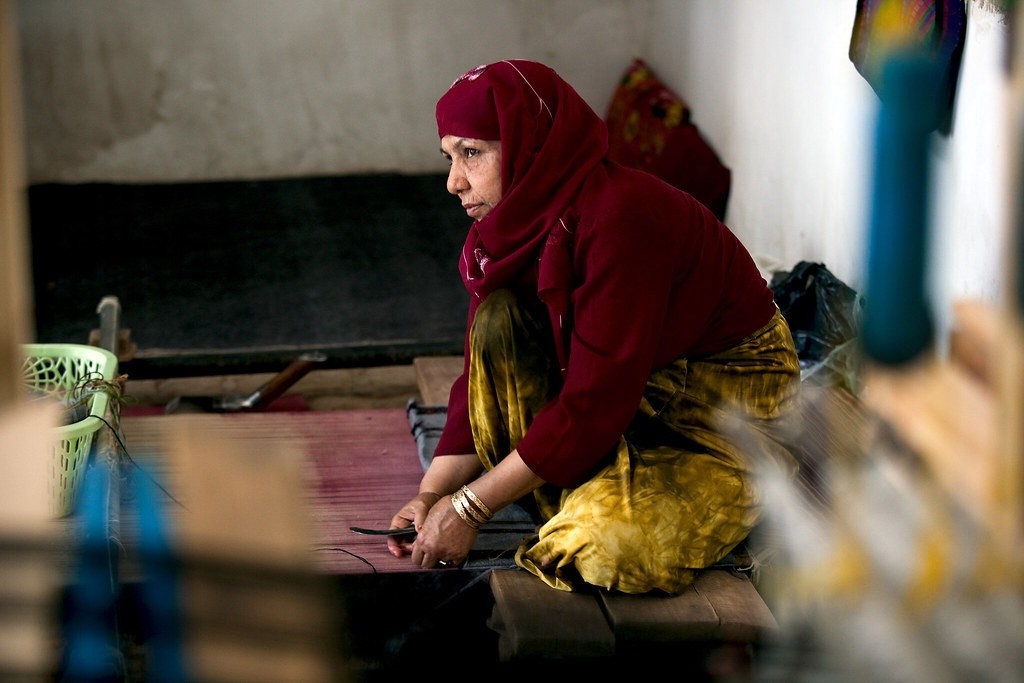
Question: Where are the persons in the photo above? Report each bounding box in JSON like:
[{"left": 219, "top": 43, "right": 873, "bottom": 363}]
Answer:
[{"left": 388, "top": 59, "right": 802, "bottom": 595}]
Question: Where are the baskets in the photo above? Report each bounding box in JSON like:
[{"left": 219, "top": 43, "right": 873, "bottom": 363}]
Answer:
[{"left": 19, "top": 344, "right": 117, "bottom": 522}]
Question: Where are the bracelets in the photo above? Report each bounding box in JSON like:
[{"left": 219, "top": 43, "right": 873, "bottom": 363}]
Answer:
[{"left": 451, "top": 485, "right": 494, "bottom": 529}]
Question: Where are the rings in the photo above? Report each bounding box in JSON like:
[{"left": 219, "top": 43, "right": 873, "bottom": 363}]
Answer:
[{"left": 439, "top": 560, "right": 450, "bottom": 567}]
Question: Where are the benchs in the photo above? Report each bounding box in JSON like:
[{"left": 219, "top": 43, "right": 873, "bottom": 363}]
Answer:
[{"left": 409, "top": 355, "right": 777, "bottom": 683}]
